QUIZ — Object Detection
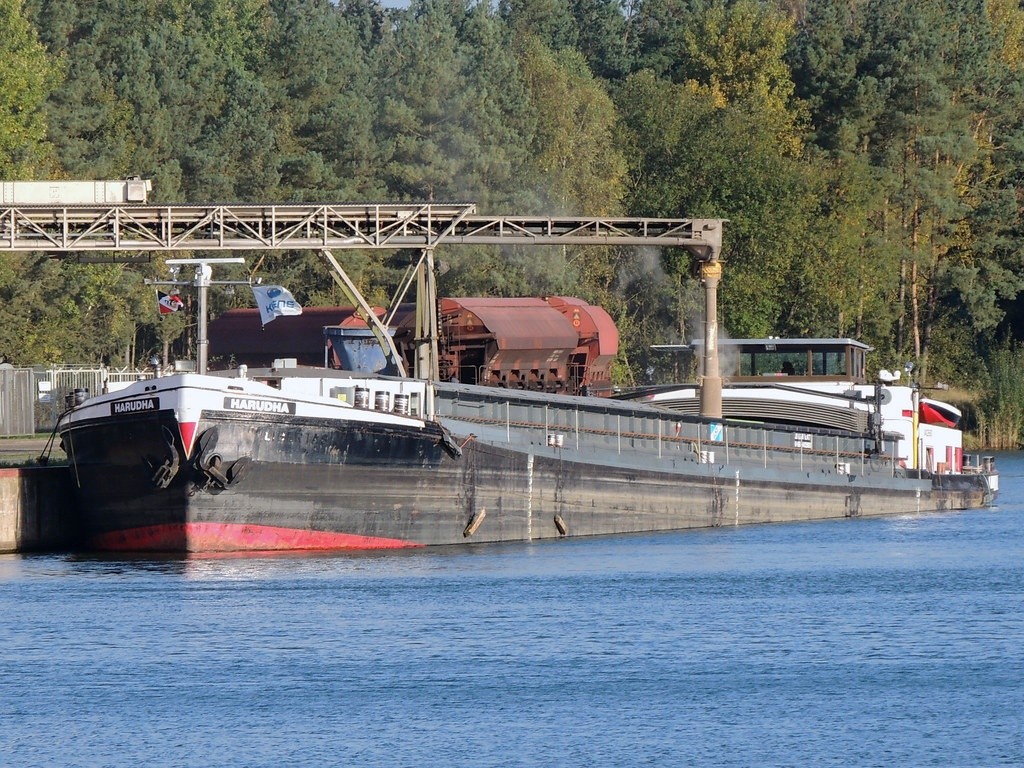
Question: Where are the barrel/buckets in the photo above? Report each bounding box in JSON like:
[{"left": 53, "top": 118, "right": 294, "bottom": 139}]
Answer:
[
  {"left": 938, "top": 462, "right": 946, "bottom": 475},
  {"left": 963, "top": 454, "right": 971, "bottom": 466},
  {"left": 394, "top": 394, "right": 409, "bottom": 414},
  {"left": 355, "top": 388, "right": 369, "bottom": 410},
  {"left": 700, "top": 451, "right": 708, "bottom": 464},
  {"left": 64, "top": 388, "right": 88, "bottom": 411},
  {"left": 838, "top": 463, "right": 845, "bottom": 475},
  {"left": 846, "top": 463, "right": 851, "bottom": 474},
  {"left": 708, "top": 452, "right": 715, "bottom": 463},
  {"left": 374, "top": 391, "right": 390, "bottom": 412},
  {"left": 547, "top": 434, "right": 563, "bottom": 447},
  {"left": 982, "top": 456, "right": 995, "bottom": 473}
]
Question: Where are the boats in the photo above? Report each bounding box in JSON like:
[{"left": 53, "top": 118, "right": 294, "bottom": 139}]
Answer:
[{"left": 54, "top": 333, "right": 1003, "bottom": 559}]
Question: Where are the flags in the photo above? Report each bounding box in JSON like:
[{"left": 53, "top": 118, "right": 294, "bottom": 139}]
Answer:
[
  {"left": 157, "top": 288, "right": 183, "bottom": 313},
  {"left": 253, "top": 285, "right": 303, "bottom": 326}
]
